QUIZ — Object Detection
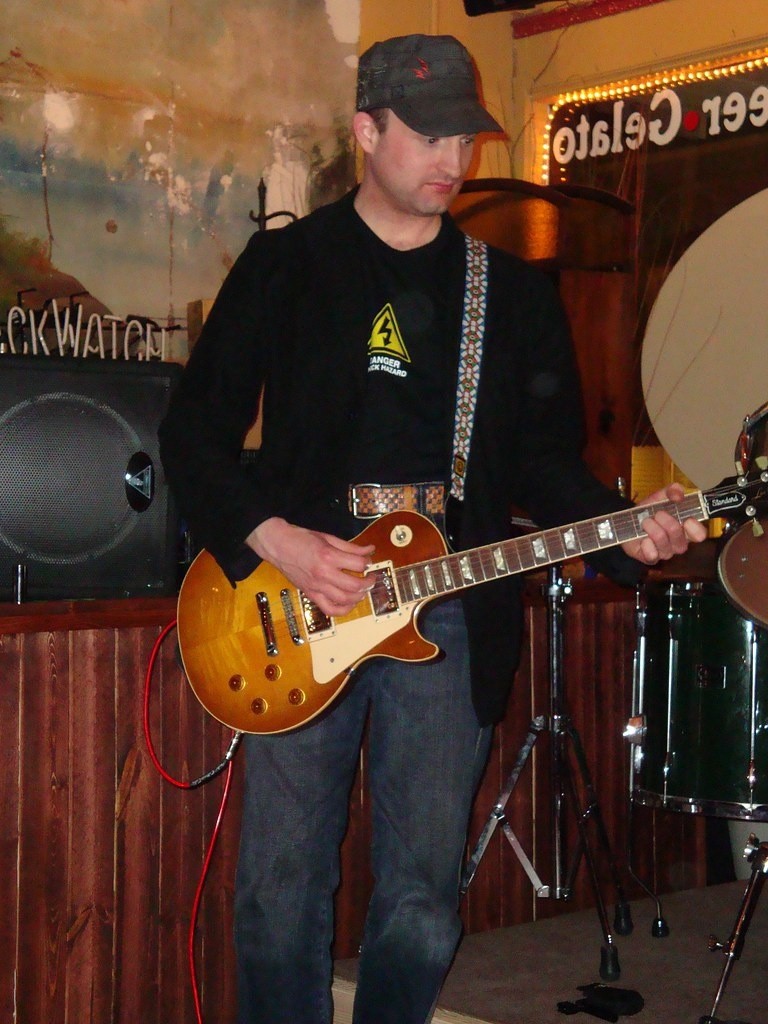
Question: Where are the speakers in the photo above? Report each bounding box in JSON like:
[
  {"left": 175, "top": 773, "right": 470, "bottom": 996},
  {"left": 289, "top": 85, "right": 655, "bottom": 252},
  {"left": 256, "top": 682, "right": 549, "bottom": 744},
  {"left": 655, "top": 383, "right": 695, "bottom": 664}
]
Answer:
[{"left": 0, "top": 352, "right": 195, "bottom": 602}]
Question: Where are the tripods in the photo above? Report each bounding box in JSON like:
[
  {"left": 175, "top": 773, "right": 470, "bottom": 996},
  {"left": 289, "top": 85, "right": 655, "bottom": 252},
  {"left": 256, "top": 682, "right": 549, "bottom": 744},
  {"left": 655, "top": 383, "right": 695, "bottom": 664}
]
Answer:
[{"left": 455, "top": 566, "right": 633, "bottom": 984}]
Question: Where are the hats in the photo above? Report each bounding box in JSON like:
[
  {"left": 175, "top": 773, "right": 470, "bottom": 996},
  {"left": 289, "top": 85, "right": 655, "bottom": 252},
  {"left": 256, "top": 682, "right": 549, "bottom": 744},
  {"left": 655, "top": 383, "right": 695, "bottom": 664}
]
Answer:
[{"left": 356, "top": 34, "right": 505, "bottom": 137}]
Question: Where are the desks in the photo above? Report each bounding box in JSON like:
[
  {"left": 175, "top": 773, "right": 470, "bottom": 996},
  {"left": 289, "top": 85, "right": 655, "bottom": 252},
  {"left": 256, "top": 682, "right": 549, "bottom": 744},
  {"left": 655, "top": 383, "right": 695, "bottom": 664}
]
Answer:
[{"left": 0, "top": 574, "right": 707, "bottom": 1024}]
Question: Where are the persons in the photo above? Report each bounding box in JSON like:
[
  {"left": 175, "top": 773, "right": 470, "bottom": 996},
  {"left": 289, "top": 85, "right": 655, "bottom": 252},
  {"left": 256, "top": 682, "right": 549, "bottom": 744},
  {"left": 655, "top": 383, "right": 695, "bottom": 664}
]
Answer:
[{"left": 157, "top": 32, "right": 710, "bottom": 1024}]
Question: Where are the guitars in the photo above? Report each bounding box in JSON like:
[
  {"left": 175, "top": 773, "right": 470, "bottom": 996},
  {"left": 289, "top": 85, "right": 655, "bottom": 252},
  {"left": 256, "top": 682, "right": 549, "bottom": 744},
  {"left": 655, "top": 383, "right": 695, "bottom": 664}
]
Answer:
[{"left": 174, "top": 464, "right": 767, "bottom": 737}]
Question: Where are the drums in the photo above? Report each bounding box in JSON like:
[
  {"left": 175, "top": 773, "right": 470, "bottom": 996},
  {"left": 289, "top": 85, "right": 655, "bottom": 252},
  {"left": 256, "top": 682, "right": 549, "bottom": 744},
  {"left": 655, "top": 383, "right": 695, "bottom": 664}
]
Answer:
[
  {"left": 732, "top": 400, "right": 768, "bottom": 537},
  {"left": 626, "top": 575, "right": 767, "bottom": 823}
]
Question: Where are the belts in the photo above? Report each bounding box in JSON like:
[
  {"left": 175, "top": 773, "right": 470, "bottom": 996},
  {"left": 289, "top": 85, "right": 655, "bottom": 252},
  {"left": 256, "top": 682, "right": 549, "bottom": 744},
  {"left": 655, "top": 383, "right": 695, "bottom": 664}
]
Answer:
[{"left": 348, "top": 481, "right": 447, "bottom": 519}]
